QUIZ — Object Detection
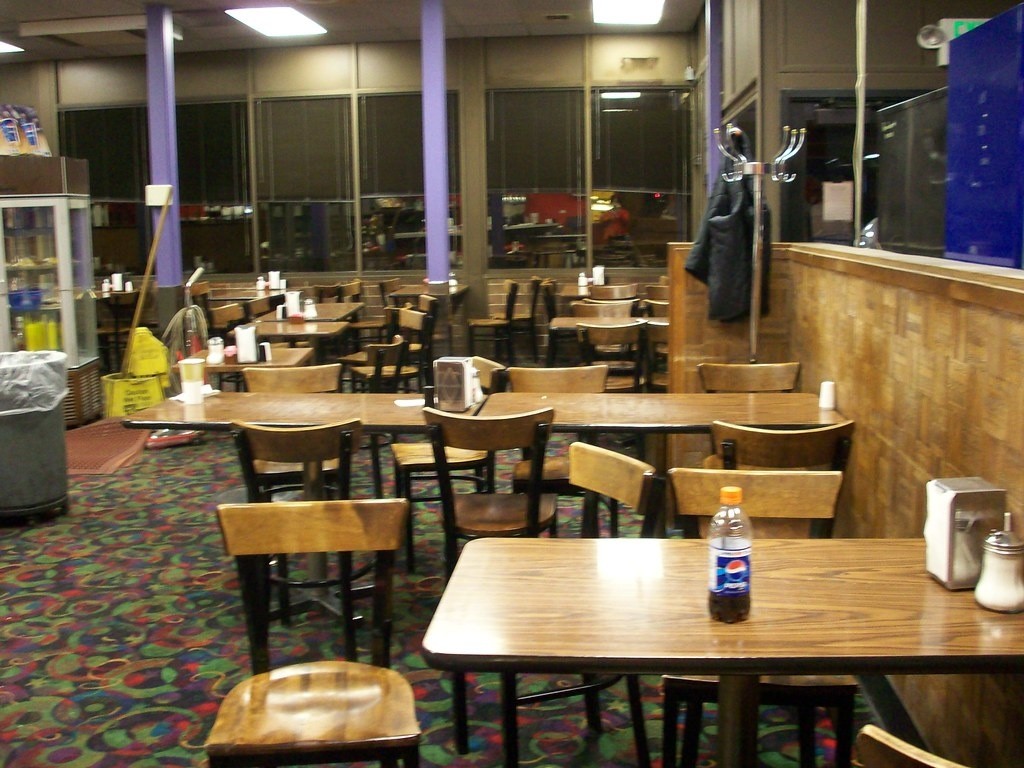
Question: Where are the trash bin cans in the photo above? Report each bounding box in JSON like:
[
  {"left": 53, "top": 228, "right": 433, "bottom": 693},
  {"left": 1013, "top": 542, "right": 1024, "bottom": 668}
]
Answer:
[{"left": 0, "top": 350, "right": 70, "bottom": 522}]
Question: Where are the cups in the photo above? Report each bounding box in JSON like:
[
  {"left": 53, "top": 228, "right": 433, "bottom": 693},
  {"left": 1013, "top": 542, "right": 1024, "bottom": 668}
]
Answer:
[
  {"left": 21, "top": 122, "right": 39, "bottom": 147},
  {"left": 0, "top": 118, "right": 21, "bottom": 145},
  {"left": 178, "top": 358, "right": 206, "bottom": 405}
]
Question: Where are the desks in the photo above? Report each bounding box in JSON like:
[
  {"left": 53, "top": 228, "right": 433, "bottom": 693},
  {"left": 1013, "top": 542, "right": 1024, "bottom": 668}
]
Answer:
[
  {"left": 255, "top": 302, "right": 365, "bottom": 322},
  {"left": 561, "top": 284, "right": 592, "bottom": 300},
  {"left": 229, "top": 321, "right": 351, "bottom": 366},
  {"left": 475, "top": 393, "right": 846, "bottom": 535},
  {"left": 171, "top": 348, "right": 315, "bottom": 505},
  {"left": 388, "top": 284, "right": 469, "bottom": 298},
  {"left": 122, "top": 392, "right": 488, "bottom": 626},
  {"left": 549, "top": 316, "right": 670, "bottom": 337},
  {"left": 393, "top": 221, "right": 558, "bottom": 269},
  {"left": 205, "top": 290, "right": 286, "bottom": 301},
  {"left": 422, "top": 536, "right": 1024, "bottom": 768}
]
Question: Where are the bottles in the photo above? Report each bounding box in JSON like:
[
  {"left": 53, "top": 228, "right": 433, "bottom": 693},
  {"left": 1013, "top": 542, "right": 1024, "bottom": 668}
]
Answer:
[
  {"left": 709, "top": 485, "right": 754, "bottom": 623},
  {"left": 207, "top": 336, "right": 224, "bottom": 364},
  {"left": 304, "top": 299, "right": 317, "bottom": 318},
  {"left": 472, "top": 368, "right": 481, "bottom": 402},
  {"left": 578, "top": 273, "right": 588, "bottom": 286},
  {"left": 256, "top": 276, "right": 266, "bottom": 290},
  {"left": 448, "top": 272, "right": 456, "bottom": 285},
  {"left": 101, "top": 279, "right": 110, "bottom": 292},
  {"left": 974, "top": 531, "right": 1024, "bottom": 613}
]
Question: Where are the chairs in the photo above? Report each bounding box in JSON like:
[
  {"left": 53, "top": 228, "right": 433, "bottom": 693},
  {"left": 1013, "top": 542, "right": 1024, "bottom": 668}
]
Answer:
[
  {"left": 595, "top": 240, "right": 669, "bottom": 268},
  {"left": 512, "top": 234, "right": 587, "bottom": 268},
  {"left": 94, "top": 279, "right": 978, "bottom": 768}
]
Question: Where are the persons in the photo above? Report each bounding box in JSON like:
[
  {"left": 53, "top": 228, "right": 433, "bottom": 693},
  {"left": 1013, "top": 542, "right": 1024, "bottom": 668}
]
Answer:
[{"left": 601, "top": 194, "right": 630, "bottom": 241}]
